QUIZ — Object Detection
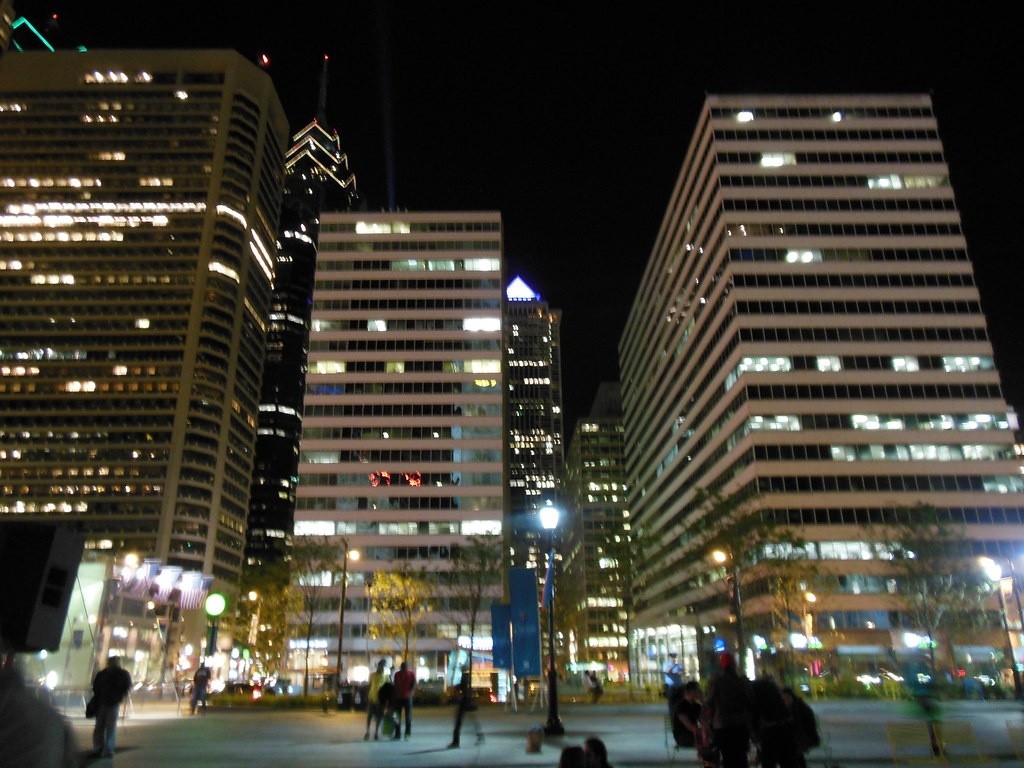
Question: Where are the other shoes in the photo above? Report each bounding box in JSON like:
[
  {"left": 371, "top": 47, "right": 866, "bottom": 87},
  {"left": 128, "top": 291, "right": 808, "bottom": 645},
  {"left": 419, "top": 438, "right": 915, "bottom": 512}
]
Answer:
[
  {"left": 448, "top": 742, "right": 458, "bottom": 749},
  {"left": 475, "top": 735, "right": 485, "bottom": 745}
]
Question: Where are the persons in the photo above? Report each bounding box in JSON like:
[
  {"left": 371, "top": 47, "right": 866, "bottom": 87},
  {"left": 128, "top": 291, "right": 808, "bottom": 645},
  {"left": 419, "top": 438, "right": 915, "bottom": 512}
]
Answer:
[
  {"left": 559, "top": 738, "right": 611, "bottom": 767},
  {"left": 365, "top": 659, "right": 416, "bottom": 741},
  {"left": 92, "top": 656, "right": 131, "bottom": 758},
  {"left": 189, "top": 664, "right": 211, "bottom": 714},
  {"left": 662, "top": 652, "right": 820, "bottom": 767},
  {"left": 583, "top": 670, "right": 604, "bottom": 705},
  {"left": 447, "top": 665, "right": 484, "bottom": 748}
]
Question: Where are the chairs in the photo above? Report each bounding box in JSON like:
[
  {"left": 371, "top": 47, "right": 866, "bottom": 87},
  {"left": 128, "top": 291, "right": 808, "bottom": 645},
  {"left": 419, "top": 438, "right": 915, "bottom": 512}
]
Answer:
[
  {"left": 884, "top": 721, "right": 939, "bottom": 768},
  {"left": 803, "top": 715, "right": 833, "bottom": 768},
  {"left": 933, "top": 720, "right": 991, "bottom": 768},
  {"left": 1007, "top": 718, "right": 1024, "bottom": 768},
  {"left": 663, "top": 713, "right": 679, "bottom": 766},
  {"left": 869, "top": 679, "right": 916, "bottom": 702}
]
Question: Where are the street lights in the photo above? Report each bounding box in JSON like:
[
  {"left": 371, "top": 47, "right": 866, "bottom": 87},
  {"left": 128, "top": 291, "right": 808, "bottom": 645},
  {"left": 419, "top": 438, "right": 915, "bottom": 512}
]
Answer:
[
  {"left": 333, "top": 535, "right": 349, "bottom": 709},
  {"left": 538, "top": 505, "right": 566, "bottom": 738},
  {"left": 978, "top": 553, "right": 1024, "bottom": 636},
  {"left": 710, "top": 540, "right": 748, "bottom": 681},
  {"left": 986, "top": 565, "right": 1024, "bottom": 701}
]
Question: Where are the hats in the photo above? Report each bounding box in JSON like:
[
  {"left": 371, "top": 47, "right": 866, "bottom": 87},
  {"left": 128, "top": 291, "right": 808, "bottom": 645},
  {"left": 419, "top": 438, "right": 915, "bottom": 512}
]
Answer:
[
  {"left": 720, "top": 653, "right": 736, "bottom": 668},
  {"left": 669, "top": 653, "right": 677, "bottom": 658}
]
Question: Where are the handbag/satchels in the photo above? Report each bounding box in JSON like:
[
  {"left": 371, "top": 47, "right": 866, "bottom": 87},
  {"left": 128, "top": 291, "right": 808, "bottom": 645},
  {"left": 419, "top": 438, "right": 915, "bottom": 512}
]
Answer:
[
  {"left": 87, "top": 696, "right": 96, "bottom": 718},
  {"left": 463, "top": 697, "right": 478, "bottom": 712},
  {"left": 382, "top": 707, "right": 394, "bottom": 736}
]
